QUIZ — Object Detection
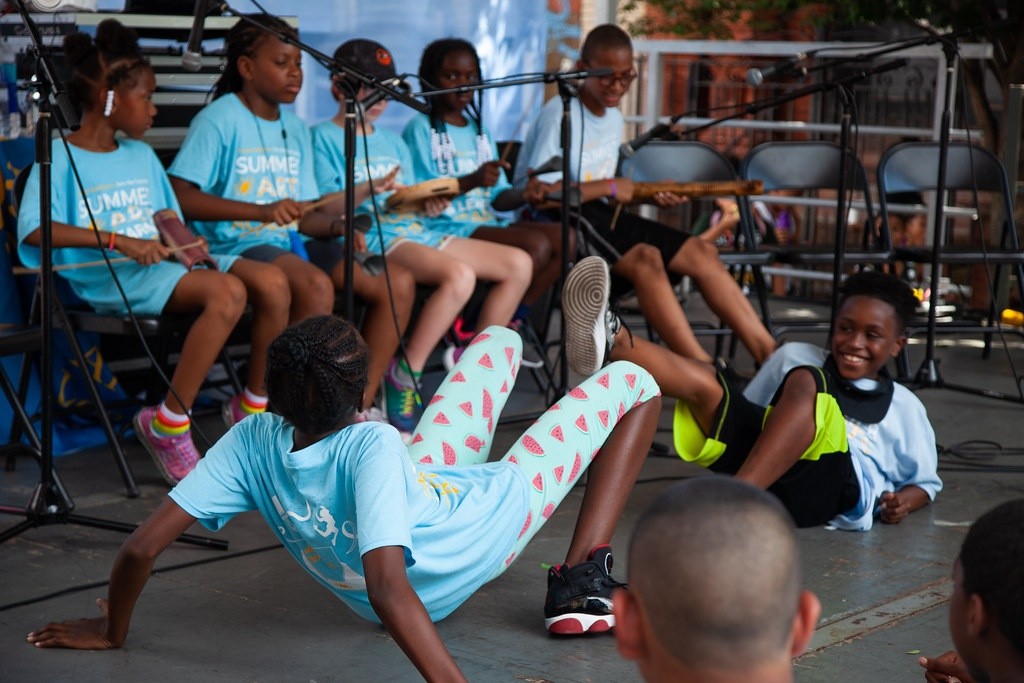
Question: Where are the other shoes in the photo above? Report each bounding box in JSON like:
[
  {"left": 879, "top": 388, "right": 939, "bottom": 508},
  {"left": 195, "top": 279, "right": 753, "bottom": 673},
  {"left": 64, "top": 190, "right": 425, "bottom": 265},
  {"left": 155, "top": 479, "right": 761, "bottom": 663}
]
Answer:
[{"left": 713, "top": 357, "right": 739, "bottom": 384}]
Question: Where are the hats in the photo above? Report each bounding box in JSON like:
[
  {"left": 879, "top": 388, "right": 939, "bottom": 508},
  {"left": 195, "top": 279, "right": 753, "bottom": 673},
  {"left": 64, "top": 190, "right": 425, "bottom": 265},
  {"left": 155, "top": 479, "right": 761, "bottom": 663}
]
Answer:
[{"left": 331, "top": 39, "right": 410, "bottom": 90}]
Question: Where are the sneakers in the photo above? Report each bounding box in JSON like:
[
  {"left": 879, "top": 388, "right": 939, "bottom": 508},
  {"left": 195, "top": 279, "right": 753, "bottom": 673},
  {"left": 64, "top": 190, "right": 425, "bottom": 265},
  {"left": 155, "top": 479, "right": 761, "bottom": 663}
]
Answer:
[
  {"left": 222, "top": 396, "right": 245, "bottom": 429},
  {"left": 508, "top": 319, "right": 544, "bottom": 367},
  {"left": 354, "top": 402, "right": 388, "bottom": 423},
  {"left": 133, "top": 404, "right": 201, "bottom": 487},
  {"left": 381, "top": 357, "right": 424, "bottom": 431},
  {"left": 561, "top": 255, "right": 634, "bottom": 378},
  {"left": 443, "top": 317, "right": 477, "bottom": 373},
  {"left": 543, "top": 543, "right": 629, "bottom": 634}
]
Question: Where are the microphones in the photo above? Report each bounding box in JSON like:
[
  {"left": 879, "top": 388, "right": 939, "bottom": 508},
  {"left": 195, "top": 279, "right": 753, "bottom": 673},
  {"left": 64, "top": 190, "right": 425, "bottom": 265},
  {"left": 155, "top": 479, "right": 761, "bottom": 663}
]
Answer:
[
  {"left": 745, "top": 47, "right": 817, "bottom": 86},
  {"left": 356, "top": 73, "right": 408, "bottom": 120},
  {"left": 912, "top": 19, "right": 953, "bottom": 46},
  {"left": 618, "top": 115, "right": 680, "bottom": 157},
  {"left": 179, "top": 0, "right": 208, "bottom": 72}
]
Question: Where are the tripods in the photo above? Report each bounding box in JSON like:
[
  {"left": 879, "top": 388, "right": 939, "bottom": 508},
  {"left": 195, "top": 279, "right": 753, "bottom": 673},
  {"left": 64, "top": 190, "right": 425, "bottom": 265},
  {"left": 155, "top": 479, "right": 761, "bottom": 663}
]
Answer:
[
  {"left": 1, "top": 1, "right": 229, "bottom": 553},
  {"left": 788, "top": 32, "right": 1024, "bottom": 405}
]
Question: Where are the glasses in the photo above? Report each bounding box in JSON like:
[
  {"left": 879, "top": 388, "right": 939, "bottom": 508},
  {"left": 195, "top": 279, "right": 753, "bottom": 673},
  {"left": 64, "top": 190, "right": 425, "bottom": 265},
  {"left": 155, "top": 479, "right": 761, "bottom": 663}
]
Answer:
[{"left": 584, "top": 61, "right": 638, "bottom": 87}]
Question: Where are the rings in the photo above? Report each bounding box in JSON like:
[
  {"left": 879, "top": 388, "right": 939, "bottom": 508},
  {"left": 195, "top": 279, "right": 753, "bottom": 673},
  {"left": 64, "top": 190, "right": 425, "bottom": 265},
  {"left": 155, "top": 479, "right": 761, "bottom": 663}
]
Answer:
[{"left": 945, "top": 676, "right": 951, "bottom": 680}]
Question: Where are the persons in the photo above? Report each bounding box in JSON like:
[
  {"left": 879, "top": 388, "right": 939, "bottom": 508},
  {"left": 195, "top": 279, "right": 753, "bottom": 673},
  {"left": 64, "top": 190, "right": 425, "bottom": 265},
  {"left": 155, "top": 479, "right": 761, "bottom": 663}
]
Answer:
[
  {"left": 562, "top": 255, "right": 943, "bottom": 531},
  {"left": 27, "top": 315, "right": 660, "bottom": 683},
  {"left": 874, "top": 191, "right": 928, "bottom": 281},
  {"left": 19, "top": 12, "right": 577, "bottom": 486},
  {"left": 919, "top": 499, "right": 1024, "bottom": 683},
  {"left": 610, "top": 476, "right": 819, "bottom": 683},
  {"left": 513, "top": 24, "right": 787, "bottom": 371}
]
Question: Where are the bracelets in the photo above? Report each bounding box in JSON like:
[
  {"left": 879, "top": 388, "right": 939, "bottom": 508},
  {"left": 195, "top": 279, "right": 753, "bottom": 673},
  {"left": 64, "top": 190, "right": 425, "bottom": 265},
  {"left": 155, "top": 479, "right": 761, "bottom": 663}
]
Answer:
[
  {"left": 109, "top": 231, "right": 115, "bottom": 251},
  {"left": 609, "top": 180, "right": 617, "bottom": 198},
  {"left": 330, "top": 220, "right": 344, "bottom": 239}
]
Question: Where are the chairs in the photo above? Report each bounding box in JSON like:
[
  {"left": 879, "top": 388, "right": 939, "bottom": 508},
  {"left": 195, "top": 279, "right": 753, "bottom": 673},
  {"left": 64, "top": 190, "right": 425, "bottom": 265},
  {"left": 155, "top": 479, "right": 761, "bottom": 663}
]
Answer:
[
  {"left": 877, "top": 141, "right": 1024, "bottom": 385},
  {"left": 0, "top": 135, "right": 772, "bottom": 514},
  {"left": 736, "top": 141, "right": 905, "bottom": 386},
  {"left": 605, "top": 142, "right": 777, "bottom": 377}
]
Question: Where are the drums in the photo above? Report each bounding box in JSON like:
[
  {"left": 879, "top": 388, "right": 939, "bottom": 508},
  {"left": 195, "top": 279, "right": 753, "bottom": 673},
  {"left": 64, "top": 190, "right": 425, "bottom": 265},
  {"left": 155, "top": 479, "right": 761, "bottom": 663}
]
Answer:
[{"left": 384, "top": 177, "right": 463, "bottom": 216}]
